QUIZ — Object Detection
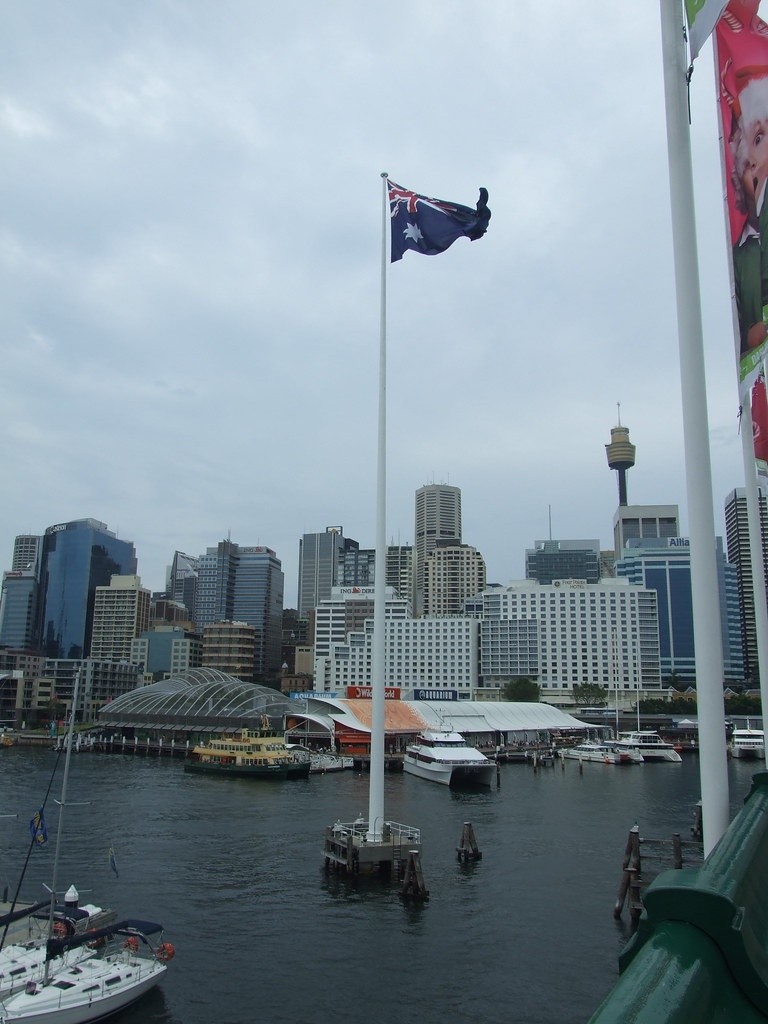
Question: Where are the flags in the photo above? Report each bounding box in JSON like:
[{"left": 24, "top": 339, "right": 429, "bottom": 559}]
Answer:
[
  {"left": 31, "top": 805, "right": 48, "bottom": 848},
  {"left": 387, "top": 178, "right": 492, "bottom": 262},
  {"left": 110, "top": 847, "right": 118, "bottom": 877}
]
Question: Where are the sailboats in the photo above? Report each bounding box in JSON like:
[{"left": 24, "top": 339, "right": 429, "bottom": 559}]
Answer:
[
  {"left": 0, "top": 668, "right": 176, "bottom": 1023},
  {"left": 617, "top": 641, "right": 683, "bottom": 763}
]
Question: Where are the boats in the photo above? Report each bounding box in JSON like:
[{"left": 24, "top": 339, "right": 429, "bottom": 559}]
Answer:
[
  {"left": 403, "top": 725, "right": 497, "bottom": 788},
  {"left": 730, "top": 728, "right": 765, "bottom": 759},
  {"left": 558, "top": 742, "right": 645, "bottom": 764},
  {"left": 183, "top": 716, "right": 311, "bottom": 781},
  {"left": 1, "top": 736, "right": 13, "bottom": 746}
]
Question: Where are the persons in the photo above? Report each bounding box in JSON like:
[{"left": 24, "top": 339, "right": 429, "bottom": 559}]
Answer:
[{"left": 728, "top": 66, "right": 768, "bottom": 359}]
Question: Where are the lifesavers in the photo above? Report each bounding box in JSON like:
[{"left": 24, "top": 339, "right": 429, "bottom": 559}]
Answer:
[
  {"left": 243, "top": 738, "right": 250, "bottom": 743},
  {"left": 123, "top": 936, "right": 139, "bottom": 954},
  {"left": 157, "top": 942, "right": 175, "bottom": 961}
]
